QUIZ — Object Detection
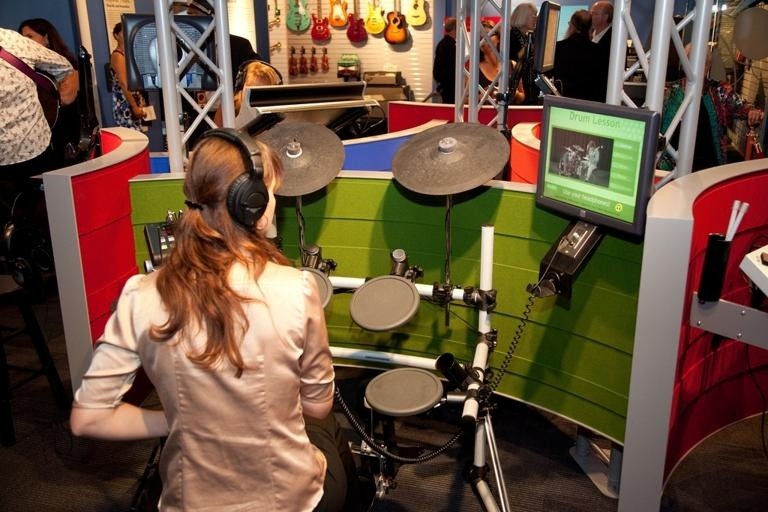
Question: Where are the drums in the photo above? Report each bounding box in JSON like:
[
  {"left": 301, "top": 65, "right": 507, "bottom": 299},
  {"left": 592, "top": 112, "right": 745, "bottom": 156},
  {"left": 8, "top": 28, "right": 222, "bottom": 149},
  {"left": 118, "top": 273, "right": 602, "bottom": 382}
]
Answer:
[
  {"left": 366, "top": 367, "right": 443, "bottom": 418},
  {"left": 299, "top": 267, "right": 333, "bottom": 309},
  {"left": 349, "top": 275, "right": 420, "bottom": 332}
]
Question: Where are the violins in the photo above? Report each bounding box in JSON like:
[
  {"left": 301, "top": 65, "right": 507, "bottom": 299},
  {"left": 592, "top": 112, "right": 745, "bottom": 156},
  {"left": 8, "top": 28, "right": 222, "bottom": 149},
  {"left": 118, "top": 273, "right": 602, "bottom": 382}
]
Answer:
[
  {"left": 322, "top": 48, "right": 329, "bottom": 71},
  {"left": 311, "top": 47, "right": 318, "bottom": 72},
  {"left": 289, "top": 46, "right": 298, "bottom": 75},
  {"left": 299, "top": 46, "right": 309, "bottom": 74}
]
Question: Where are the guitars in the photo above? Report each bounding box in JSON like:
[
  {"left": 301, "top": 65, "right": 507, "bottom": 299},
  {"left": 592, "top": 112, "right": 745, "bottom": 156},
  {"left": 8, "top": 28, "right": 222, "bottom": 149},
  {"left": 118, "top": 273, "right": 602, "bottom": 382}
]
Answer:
[
  {"left": 347, "top": 1, "right": 367, "bottom": 42},
  {"left": 287, "top": 0, "right": 310, "bottom": 33},
  {"left": 384, "top": 0, "right": 409, "bottom": 44},
  {"left": 329, "top": 1, "right": 348, "bottom": 27},
  {"left": 365, "top": 0, "right": 385, "bottom": 35},
  {"left": 35, "top": 71, "right": 61, "bottom": 130},
  {"left": 406, "top": 0, "right": 427, "bottom": 26},
  {"left": 311, "top": 0, "right": 331, "bottom": 43}
]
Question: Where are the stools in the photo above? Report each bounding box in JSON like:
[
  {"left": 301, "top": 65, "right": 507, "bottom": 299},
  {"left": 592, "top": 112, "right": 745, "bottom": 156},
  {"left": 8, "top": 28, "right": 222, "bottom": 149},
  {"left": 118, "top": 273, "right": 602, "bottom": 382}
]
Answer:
[{"left": 0, "top": 180, "right": 71, "bottom": 448}]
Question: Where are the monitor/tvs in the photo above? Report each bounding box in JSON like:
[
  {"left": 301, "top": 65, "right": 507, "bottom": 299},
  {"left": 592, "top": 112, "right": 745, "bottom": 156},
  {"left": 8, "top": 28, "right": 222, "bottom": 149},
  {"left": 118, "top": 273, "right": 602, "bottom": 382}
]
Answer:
[
  {"left": 534, "top": 1, "right": 561, "bottom": 73},
  {"left": 534, "top": 93, "right": 661, "bottom": 237},
  {"left": 121, "top": 13, "right": 217, "bottom": 92},
  {"left": 78, "top": 45, "right": 93, "bottom": 123}
]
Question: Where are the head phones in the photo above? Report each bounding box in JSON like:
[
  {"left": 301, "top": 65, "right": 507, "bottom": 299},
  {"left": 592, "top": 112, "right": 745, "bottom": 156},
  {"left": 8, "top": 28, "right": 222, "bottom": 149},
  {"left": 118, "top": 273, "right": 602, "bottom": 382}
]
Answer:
[
  {"left": 235, "top": 58, "right": 284, "bottom": 90},
  {"left": 196, "top": 127, "right": 269, "bottom": 227},
  {"left": 3, "top": 191, "right": 52, "bottom": 288}
]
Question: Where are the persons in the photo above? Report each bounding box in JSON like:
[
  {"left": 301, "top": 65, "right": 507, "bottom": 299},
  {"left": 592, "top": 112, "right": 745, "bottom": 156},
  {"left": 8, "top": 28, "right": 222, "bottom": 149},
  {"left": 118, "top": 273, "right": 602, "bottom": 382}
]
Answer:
[
  {"left": 577, "top": 141, "right": 600, "bottom": 181},
  {"left": 70, "top": 137, "right": 356, "bottom": 512},
  {"left": 19, "top": 18, "right": 100, "bottom": 167},
  {"left": 110, "top": 22, "right": 147, "bottom": 133},
  {"left": 182, "top": 0, "right": 262, "bottom": 159},
  {"left": 0, "top": 26, "right": 75, "bottom": 258},
  {"left": 214, "top": 60, "right": 278, "bottom": 131},
  {"left": 433, "top": 0, "right": 767, "bottom": 174}
]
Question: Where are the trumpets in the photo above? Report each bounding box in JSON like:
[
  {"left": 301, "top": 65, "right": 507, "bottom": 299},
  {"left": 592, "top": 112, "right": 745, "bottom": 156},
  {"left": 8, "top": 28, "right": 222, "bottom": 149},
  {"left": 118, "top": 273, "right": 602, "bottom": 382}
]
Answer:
[
  {"left": 270, "top": 42, "right": 280, "bottom": 54},
  {"left": 268, "top": 17, "right": 280, "bottom": 31}
]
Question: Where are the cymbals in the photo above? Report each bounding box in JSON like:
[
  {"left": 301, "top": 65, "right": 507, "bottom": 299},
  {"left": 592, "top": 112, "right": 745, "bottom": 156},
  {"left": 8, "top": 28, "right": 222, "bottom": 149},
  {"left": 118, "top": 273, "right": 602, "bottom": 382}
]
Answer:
[
  {"left": 253, "top": 121, "right": 344, "bottom": 197},
  {"left": 393, "top": 121, "right": 510, "bottom": 199}
]
